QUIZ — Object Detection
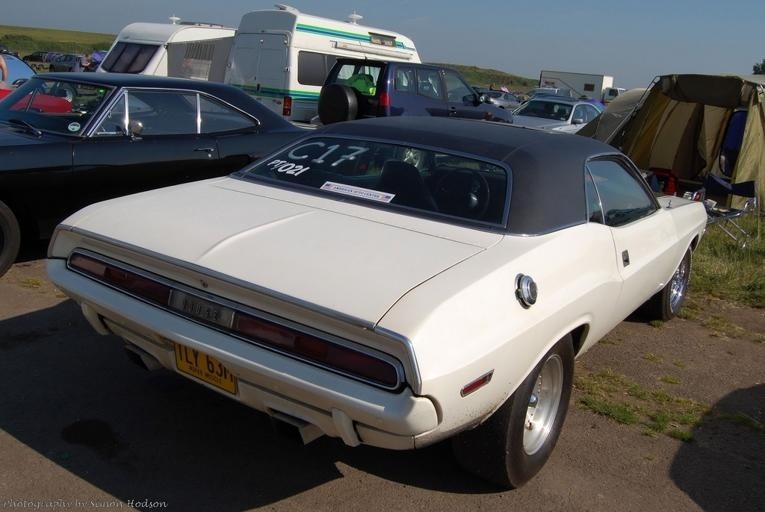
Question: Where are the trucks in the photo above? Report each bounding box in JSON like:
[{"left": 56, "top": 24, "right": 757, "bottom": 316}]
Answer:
[
  {"left": 223, "top": 4, "right": 423, "bottom": 124},
  {"left": 96, "top": 12, "right": 237, "bottom": 82},
  {"left": 539, "top": 70, "right": 626, "bottom": 107}
]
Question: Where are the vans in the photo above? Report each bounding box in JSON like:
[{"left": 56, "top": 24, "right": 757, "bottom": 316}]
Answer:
[{"left": 50, "top": 53, "right": 86, "bottom": 72}]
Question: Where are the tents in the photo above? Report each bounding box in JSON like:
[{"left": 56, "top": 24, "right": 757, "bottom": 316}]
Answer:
[{"left": 564, "top": 73, "right": 764, "bottom": 215}]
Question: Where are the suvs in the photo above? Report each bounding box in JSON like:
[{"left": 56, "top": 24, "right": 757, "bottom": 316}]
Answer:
[
  {"left": 22, "top": 51, "right": 49, "bottom": 64},
  {"left": 320, "top": 56, "right": 515, "bottom": 134}
]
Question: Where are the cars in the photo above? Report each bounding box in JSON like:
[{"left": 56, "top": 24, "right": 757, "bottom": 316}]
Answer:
[
  {"left": 509, "top": 93, "right": 605, "bottom": 137},
  {"left": 1, "top": 50, "right": 48, "bottom": 99},
  {"left": 1, "top": 68, "right": 317, "bottom": 287},
  {"left": 473, "top": 84, "right": 573, "bottom": 109}
]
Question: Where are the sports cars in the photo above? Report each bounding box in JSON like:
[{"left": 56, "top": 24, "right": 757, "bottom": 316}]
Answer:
[{"left": 45, "top": 117, "right": 709, "bottom": 488}]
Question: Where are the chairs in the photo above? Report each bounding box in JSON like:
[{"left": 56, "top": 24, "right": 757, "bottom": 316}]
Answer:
[
  {"left": 420, "top": 80, "right": 436, "bottom": 98},
  {"left": 690, "top": 170, "right": 761, "bottom": 250},
  {"left": 379, "top": 159, "right": 442, "bottom": 216},
  {"left": 348, "top": 72, "right": 373, "bottom": 93}
]
